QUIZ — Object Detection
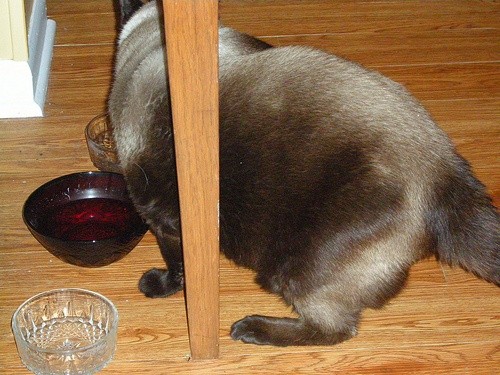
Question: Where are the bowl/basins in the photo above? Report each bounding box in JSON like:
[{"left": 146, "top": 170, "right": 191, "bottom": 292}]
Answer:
[
  {"left": 10, "top": 285, "right": 120, "bottom": 375},
  {"left": 21, "top": 170, "right": 150, "bottom": 267},
  {"left": 84, "top": 112, "right": 129, "bottom": 173}
]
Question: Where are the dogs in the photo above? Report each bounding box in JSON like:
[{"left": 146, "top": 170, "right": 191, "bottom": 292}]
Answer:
[{"left": 112, "top": 0, "right": 499, "bottom": 347}]
